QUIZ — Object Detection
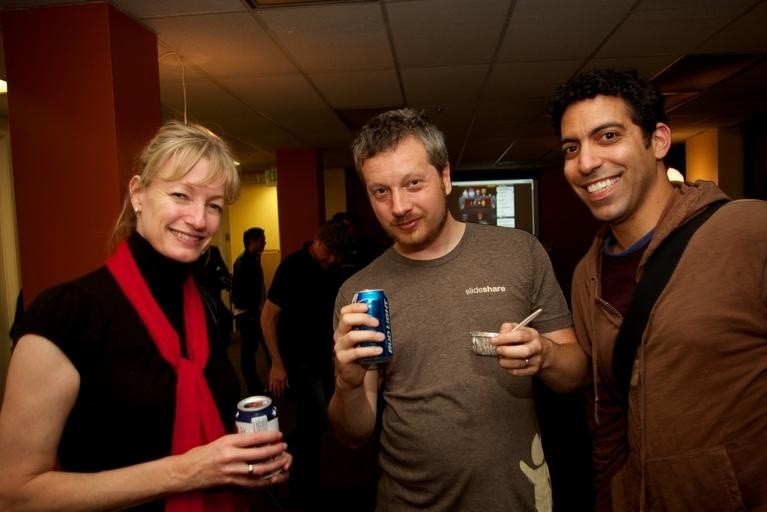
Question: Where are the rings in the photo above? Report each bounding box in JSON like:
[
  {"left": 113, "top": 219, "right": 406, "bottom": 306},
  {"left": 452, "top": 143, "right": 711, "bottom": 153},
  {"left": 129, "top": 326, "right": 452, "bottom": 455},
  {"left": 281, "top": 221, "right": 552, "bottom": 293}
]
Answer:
[
  {"left": 248, "top": 464, "right": 252, "bottom": 475},
  {"left": 524, "top": 358, "right": 528, "bottom": 367}
]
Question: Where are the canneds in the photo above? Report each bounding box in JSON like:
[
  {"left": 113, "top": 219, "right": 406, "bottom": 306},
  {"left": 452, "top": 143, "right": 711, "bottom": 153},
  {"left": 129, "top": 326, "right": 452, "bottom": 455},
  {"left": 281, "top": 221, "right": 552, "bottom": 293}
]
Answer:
[
  {"left": 235, "top": 396, "right": 283, "bottom": 480},
  {"left": 352, "top": 288, "right": 393, "bottom": 364}
]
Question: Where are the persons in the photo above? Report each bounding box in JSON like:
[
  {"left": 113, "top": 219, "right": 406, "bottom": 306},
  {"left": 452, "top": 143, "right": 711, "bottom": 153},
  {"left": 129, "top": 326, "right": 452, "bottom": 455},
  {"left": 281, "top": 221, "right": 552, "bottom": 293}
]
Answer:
[
  {"left": 326, "top": 107, "right": 589, "bottom": 512},
  {"left": 191, "top": 246, "right": 231, "bottom": 302},
  {"left": 260, "top": 219, "right": 347, "bottom": 512},
  {"left": 0, "top": 118, "right": 302, "bottom": 510},
  {"left": 230, "top": 227, "right": 266, "bottom": 393},
  {"left": 547, "top": 67, "right": 765, "bottom": 510},
  {"left": 337, "top": 213, "right": 361, "bottom": 282}
]
